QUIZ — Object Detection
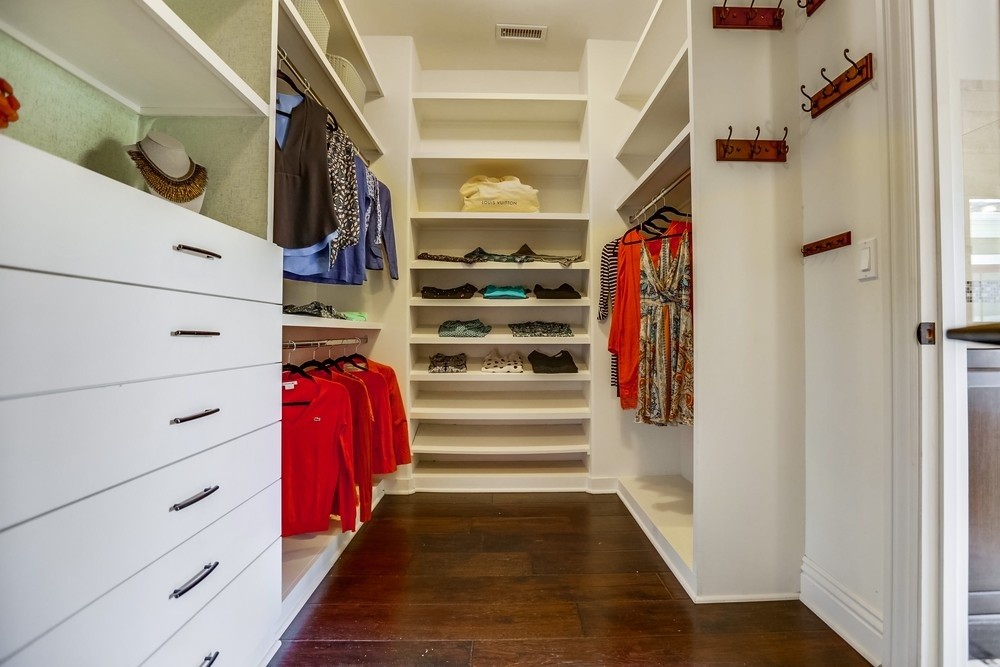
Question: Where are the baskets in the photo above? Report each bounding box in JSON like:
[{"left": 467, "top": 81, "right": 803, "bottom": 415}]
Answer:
[{"left": 290, "top": 0, "right": 367, "bottom": 117}]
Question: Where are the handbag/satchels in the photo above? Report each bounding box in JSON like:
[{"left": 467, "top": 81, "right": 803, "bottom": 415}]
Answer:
[{"left": 460, "top": 175, "right": 540, "bottom": 214}]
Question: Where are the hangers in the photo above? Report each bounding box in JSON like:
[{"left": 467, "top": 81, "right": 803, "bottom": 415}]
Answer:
[
  {"left": 282, "top": 336, "right": 368, "bottom": 406},
  {"left": 612, "top": 187, "right": 692, "bottom": 256}
]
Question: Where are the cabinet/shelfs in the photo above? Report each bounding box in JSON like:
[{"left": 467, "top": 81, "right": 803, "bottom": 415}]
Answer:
[
  {"left": 610, "top": 0, "right": 698, "bottom": 603},
  {"left": 0, "top": 0, "right": 285, "bottom": 667},
  {"left": 277, "top": 0, "right": 394, "bottom": 615},
  {"left": 404, "top": 36, "right": 594, "bottom": 482}
]
